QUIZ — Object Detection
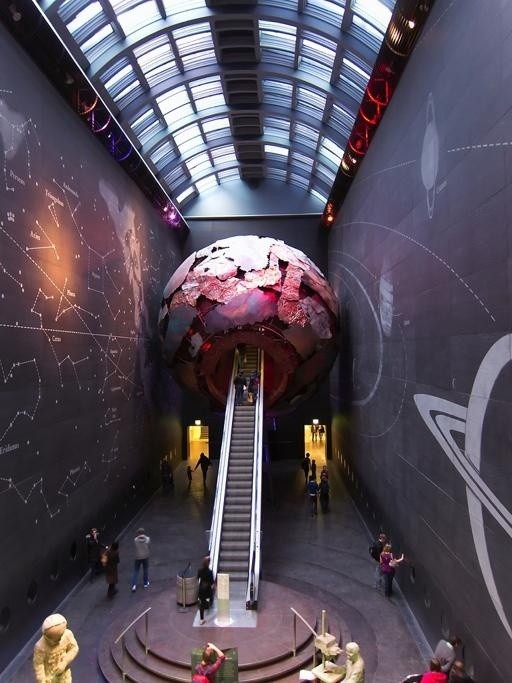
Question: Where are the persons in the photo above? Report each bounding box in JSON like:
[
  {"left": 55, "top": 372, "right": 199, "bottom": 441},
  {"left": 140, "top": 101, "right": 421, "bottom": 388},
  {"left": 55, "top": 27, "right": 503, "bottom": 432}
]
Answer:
[
  {"left": 103, "top": 540, "right": 121, "bottom": 597},
  {"left": 420, "top": 656, "right": 450, "bottom": 682},
  {"left": 248, "top": 369, "right": 261, "bottom": 405},
  {"left": 196, "top": 554, "right": 215, "bottom": 625},
  {"left": 311, "top": 424, "right": 319, "bottom": 442},
  {"left": 318, "top": 425, "right": 325, "bottom": 442},
  {"left": 31, "top": 612, "right": 80, "bottom": 682},
  {"left": 320, "top": 640, "right": 366, "bottom": 682},
  {"left": 194, "top": 452, "right": 213, "bottom": 483},
  {"left": 433, "top": 635, "right": 463, "bottom": 673},
  {"left": 192, "top": 641, "right": 226, "bottom": 682},
  {"left": 445, "top": 659, "right": 473, "bottom": 682},
  {"left": 374, "top": 532, "right": 388, "bottom": 591},
  {"left": 129, "top": 527, "right": 153, "bottom": 592},
  {"left": 186, "top": 465, "right": 194, "bottom": 483},
  {"left": 300, "top": 451, "right": 330, "bottom": 517},
  {"left": 158, "top": 459, "right": 173, "bottom": 495},
  {"left": 84, "top": 526, "right": 110, "bottom": 584},
  {"left": 233, "top": 368, "right": 248, "bottom": 405},
  {"left": 377, "top": 543, "right": 405, "bottom": 598}
]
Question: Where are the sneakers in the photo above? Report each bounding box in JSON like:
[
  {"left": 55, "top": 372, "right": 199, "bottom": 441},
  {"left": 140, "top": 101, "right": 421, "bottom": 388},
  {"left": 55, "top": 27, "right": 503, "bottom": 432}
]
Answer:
[
  {"left": 144, "top": 581, "right": 150, "bottom": 588},
  {"left": 132, "top": 584, "right": 137, "bottom": 592},
  {"left": 200, "top": 620, "right": 205, "bottom": 624}
]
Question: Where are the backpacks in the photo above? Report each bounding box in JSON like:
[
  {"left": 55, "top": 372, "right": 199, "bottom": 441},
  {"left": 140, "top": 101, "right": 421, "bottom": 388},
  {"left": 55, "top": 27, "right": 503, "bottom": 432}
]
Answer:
[{"left": 192, "top": 665, "right": 209, "bottom": 683}]
